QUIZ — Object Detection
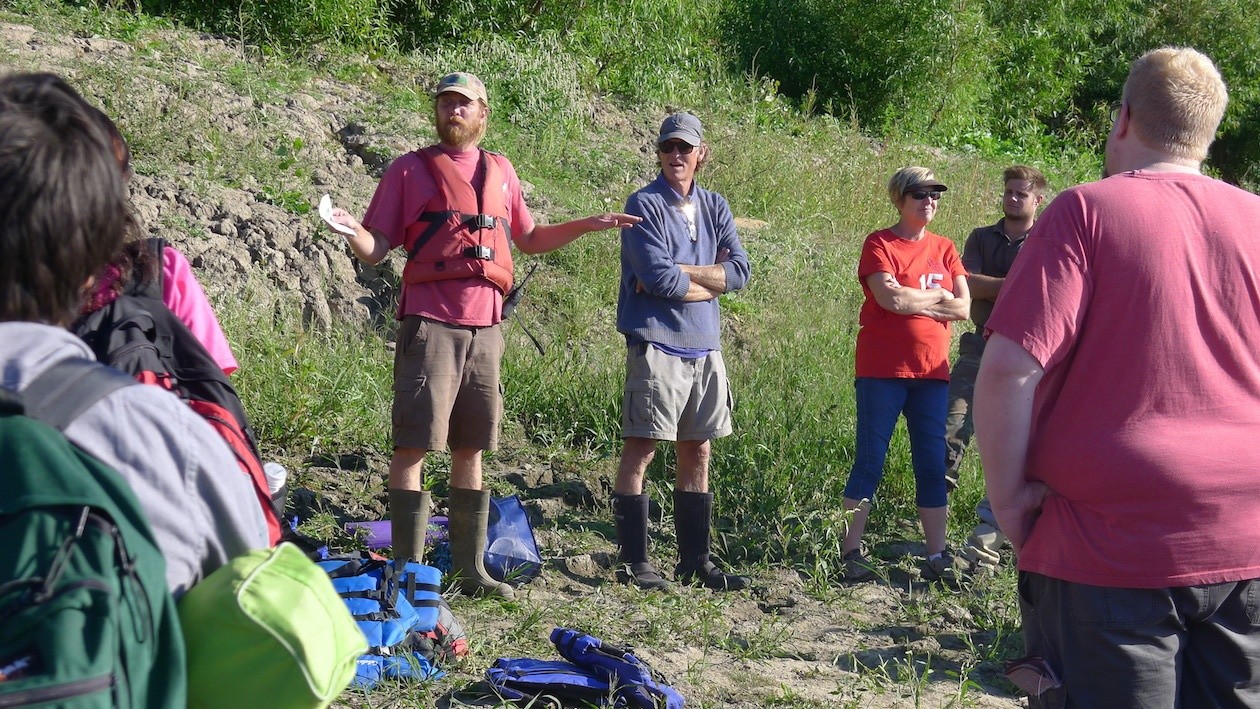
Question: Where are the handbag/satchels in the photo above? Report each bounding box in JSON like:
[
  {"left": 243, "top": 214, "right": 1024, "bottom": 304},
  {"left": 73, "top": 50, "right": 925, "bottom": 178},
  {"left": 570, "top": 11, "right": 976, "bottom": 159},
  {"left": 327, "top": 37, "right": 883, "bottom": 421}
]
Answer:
[
  {"left": 176, "top": 537, "right": 370, "bottom": 709},
  {"left": 436, "top": 493, "right": 543, "bottom": 585}
]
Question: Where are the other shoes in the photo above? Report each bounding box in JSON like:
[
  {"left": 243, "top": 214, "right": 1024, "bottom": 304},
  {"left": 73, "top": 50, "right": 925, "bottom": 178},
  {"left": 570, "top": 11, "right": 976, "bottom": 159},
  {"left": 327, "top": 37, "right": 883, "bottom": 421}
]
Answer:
[
  {"left": 926, "top": 550, "right": 964, "bottom": 587},
  {"left": 839, "top": 548, "right": 882, "bottom": 581}
]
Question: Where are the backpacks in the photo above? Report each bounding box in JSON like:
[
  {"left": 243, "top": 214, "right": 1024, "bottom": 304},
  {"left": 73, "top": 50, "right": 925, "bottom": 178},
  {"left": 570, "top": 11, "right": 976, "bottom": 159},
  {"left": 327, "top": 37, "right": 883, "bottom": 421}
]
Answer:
[
  {"left": 0, "top": 355, "right": 189, "bottom": 709},
  {"left": 485, "top": 628, "right": 685, "bottom": 709},
  {"left": 307, "top": 543, "right": 467, "bottom": 691},
  {"left": 64, "top": 238, "right": 285, "bottom": 549}
]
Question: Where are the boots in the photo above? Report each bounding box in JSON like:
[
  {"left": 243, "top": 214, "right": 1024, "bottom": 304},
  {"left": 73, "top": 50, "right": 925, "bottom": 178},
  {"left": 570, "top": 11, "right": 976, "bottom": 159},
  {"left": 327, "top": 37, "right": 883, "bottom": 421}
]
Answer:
[
  {"left": 386, "top": 487, "right": 431, "bottom": 562},
  {"left": 672, "top": 487, "right": 752, "bottom": 590},
  {"left": 612, "top": 490, "right": 669, "bottom": 591},
  {"left": 447, "top": 484, "right": 515, "bottom": 600}
]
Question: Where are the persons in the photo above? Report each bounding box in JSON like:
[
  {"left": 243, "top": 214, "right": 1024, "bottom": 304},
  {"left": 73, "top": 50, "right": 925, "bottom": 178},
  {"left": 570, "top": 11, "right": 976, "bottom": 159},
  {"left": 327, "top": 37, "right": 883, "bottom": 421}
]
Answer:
[
  {"left": 612, "top": 114, "right": 751, "bottom": 590},
  {"left": 945, "top": 165, "right": 1047, "bottom": 571},
  {"left": 837, "top": 166, "right": 971, "bottom": 585},
  {"left": 0, "top": 71, "right": 270, "bottom": 606},
  {"left": 329, "top": 73, "right": 643, "bottom": 601},
  {"left": 972, "top": 46, "right": 1260, "bottom": 709}
]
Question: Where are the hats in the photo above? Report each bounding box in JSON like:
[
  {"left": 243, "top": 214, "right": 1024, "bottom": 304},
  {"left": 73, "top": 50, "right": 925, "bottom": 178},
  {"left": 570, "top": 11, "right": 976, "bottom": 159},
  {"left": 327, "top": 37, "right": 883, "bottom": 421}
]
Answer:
[
  {"left": 904, "top": 179, "right": 948, "bottom": 192},
  {"left": 433, "top": 72, "right": 488, "bottom": 103},
  {"left": 655, "top": 113, "right": 702, "bottom": 147}
]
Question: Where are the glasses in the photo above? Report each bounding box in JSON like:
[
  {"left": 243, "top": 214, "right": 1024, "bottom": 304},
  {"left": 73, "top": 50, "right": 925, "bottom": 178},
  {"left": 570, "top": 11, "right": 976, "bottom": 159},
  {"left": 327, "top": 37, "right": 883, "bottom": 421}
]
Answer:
[
  {"left": 658, "top": 139, "right": 693, "bottom": 154},
  {"left": 676, "top": 204, "right": 697, "bottom": 242},
  {"left": 907, "top": 189, "right": 941, "bottom": 201},
  {"left": 1109, "top": 103, "right": 1131, "bottom": 122}
]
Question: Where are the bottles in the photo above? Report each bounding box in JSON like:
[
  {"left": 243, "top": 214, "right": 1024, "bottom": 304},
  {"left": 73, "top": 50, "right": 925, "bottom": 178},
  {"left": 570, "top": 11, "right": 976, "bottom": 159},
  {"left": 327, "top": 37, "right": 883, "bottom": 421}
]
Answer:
[{"left": 263, "top": 462, "right": 286, "bottom": 517}]
